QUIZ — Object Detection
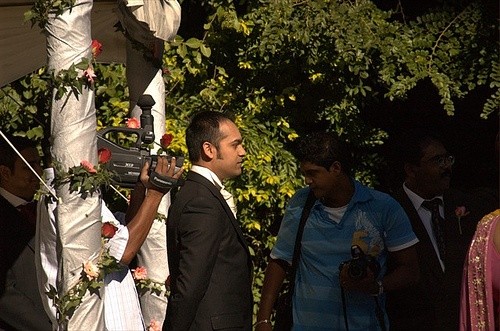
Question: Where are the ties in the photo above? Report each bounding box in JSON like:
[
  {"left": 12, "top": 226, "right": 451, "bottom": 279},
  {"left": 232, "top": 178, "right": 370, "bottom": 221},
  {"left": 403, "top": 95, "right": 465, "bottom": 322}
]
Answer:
[
  {"left": 422, "top": 198, "right": 448, "bottom": 277},
  {"left": 220, "top": 189, "right": 236, "bottom": 219},
  {"left": 16, "top": 203, "right": 36, "bottom": 225}
]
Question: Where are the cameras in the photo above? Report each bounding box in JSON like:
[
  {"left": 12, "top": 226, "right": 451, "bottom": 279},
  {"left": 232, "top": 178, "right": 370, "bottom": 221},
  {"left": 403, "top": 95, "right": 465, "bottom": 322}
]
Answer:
[{"left": 339, "top": 245, "right": 376, "bottom": 280}]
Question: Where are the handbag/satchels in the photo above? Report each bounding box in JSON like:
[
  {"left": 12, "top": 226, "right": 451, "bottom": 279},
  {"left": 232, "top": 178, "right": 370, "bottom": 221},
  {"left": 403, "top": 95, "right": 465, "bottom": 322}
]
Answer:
[{"left": 273, "top": 294, "right": 291, "bottom": 331}]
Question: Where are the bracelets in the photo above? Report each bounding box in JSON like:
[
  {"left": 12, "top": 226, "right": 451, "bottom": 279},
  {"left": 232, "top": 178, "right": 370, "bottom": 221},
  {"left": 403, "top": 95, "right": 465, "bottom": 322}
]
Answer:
[
  {"left": 371, "top": 281, "right": 384, "bottom": 297},
  {"left": 254, "top": 319, "right": 271, "bottom": 326}
]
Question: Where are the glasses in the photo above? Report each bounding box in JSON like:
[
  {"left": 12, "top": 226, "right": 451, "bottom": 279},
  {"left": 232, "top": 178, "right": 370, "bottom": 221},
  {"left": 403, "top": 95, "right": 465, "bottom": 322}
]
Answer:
[{"left": 414, "top": 156, "right": 455, "bottom": 168}]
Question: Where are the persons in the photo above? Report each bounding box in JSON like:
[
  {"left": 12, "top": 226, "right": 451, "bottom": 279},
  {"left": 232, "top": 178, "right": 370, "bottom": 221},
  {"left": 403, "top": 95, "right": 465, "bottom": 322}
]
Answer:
[
  {"left": 0, "top": 134, "right": 186, "bottom": 331},
  {"left": 162, "top": 110, "right": 254, "bottom": 331},
  {"left": 255, "top": 136, "right": 420, "bottom": 331},
  {"left": 385, "top": 136, "right": 471, "bottom": 331},
  {"left": 459, "top": 208, "right": 500, "bottom": 331}
]
